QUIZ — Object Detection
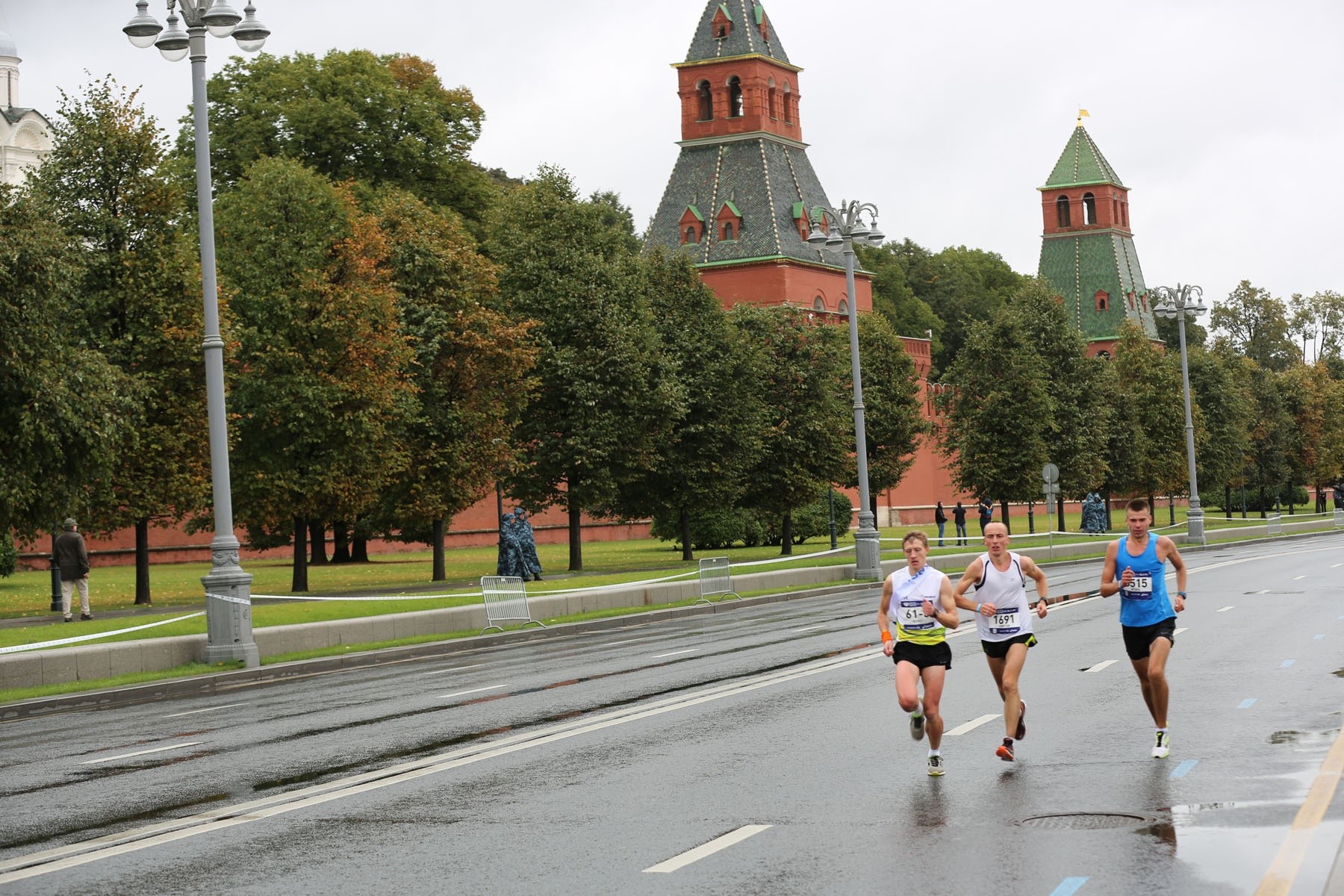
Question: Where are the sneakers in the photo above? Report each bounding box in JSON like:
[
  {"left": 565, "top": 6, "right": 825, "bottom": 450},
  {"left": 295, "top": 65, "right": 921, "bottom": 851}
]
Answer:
[
  {"left": 1014, "top": 698, "right": 1027, "bottom": 741},
  {"left": 1151, "top": 728, "right": 1171, "bottom": 757},
  {"left": 909, "top": 695, "right": 925, "bottom": 740},
  {"left": 926, "top": 757, "right": 946, "bottom": 775},
  {"left": 995, "top": 737, "right": 1015, "bottom": 762}
]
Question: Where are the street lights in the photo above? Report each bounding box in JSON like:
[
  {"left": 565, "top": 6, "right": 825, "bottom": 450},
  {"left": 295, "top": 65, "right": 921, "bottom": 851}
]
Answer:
[
  {"left": 121, "top": 0, "right": 271, "bottom": 669},
  {"left": 806, "top": 199, "right": 884, "bottom": 582},
  {"left": 1153, "top": 283, "right": 1208, "bottom": 544}
]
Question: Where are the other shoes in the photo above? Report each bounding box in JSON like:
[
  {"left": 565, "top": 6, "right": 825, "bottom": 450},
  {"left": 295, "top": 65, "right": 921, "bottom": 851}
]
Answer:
[
  {"left": 1101, "top": 531, "right": 1105, "bottom": 536},
  {"left": 1095, "top": 532, "right": 1100, "bottom": 536},
  {"left": 1088, "top": 531, "right": 1091, "bottom": 533},
  {"left": 964, "top": 544, "right": 969, "bottom": 546},
  {"left": 64, "top": 618, "right": 72, "bottom": 622},
  {"left": 80, "top": 614, "right": 94, "bottom": 620},
  {"left": 938, "top": 544, "right": 946, "bottom": 546},
  {"left": 523, "top": 578, "right": 531, "bottom": 581},
  {"left": 534, "top": 576, "right": 543, "bottom": 580},
  {"left": 956, "top": 544, "right": 961, "bottom": 546}
]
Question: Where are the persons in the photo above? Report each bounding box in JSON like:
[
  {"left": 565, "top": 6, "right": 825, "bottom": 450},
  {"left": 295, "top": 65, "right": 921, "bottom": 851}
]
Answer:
[
  {"left": 935, "top": 501, "right": 949, "bottom": 547},
  {"left": 954, "top": 521, "right": 1048, "bottom": 762},
  {"left": 496, "top": 507, "right": 543, "bottom": 583},
  {"left": 1079, "top": 492, "right": 1107, "bottom": 536},
  {"left": 877, "top": 528, "right": 959, "bottom": 777},
  {"left": 978, "top": 498, "right": 994, "bottom": 545},
  {"left": 1101, "top": 499, "right": 1187, "bottom": 760},
  {"left": 1333, "top": 488, "right": 1342, "bottom": 508},
  {"left": 1319, "top": 488, "right": 1326, "bottom": 517},
  {"left": 53, "top": 517, "right": 95, "bottom": 623},
  {"left": 951, "top": 502, "right": 969, "bottom": 546}
]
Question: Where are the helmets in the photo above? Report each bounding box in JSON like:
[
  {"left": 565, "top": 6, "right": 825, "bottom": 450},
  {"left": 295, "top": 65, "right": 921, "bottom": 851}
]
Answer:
[
  {"left": 501, "top": 513, "right": 517, "bottom": 521},
  {"left": 514, "top": 508, "right": 526, "bottom": 515}
]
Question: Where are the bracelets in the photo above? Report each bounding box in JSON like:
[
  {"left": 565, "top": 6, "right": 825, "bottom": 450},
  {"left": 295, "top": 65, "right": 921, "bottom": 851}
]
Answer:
[
  {"left": 1040, "top": 596, "right": 1048, "bottom": 607},
  {"left": 1178, "top": 592, "right": 1187, "bottom": 599},
  {"left": 976, "top": 603, "right": 982, "bottom": 613},
  {"left": 1118, "top": 580, "right": 1123, "bottom": 592},
  {"left": 931, "top": 608, "right": 939, "bottom": 618},
  {"left": 881, "top": 631, "right": 893, "bottom": 645}
]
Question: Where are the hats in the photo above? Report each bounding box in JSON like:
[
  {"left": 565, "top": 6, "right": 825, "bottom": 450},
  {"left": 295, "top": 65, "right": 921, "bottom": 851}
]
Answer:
[{"left": 64, "top": 518, "right": 81, "bottom": 526}]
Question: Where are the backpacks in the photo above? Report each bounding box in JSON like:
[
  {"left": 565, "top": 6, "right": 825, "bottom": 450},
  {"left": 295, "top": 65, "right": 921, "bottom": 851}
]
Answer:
[{"left": 983, "top": 504, "right": 993, "bottom": 519}]
